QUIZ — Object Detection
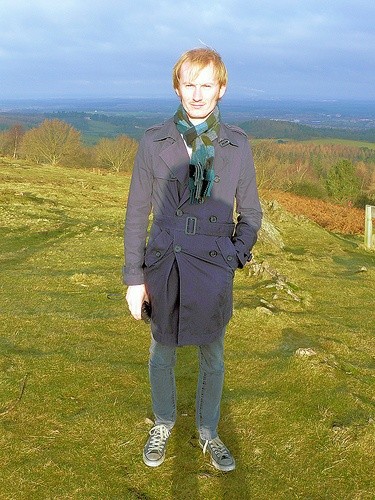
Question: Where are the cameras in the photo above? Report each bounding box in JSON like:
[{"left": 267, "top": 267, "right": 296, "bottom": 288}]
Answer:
[{"left": 141, "top": 301, "right": 152, "bottom": 322}]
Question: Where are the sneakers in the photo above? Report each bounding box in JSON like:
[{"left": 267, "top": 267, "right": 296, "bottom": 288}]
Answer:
[
  {"left": 143, "top": 424, "right": 171, "bottom": 466},
  {"left": 198, "top": 434, "right": 235, "bottom": 471}
]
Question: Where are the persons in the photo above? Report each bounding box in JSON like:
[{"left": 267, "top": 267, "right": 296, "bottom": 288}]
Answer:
[{"left": 124, "top": 48, "right": 263, "bottom": 472}]
